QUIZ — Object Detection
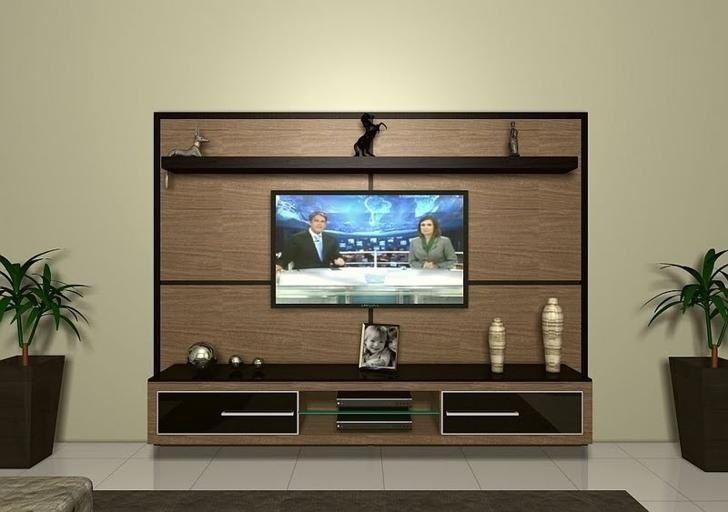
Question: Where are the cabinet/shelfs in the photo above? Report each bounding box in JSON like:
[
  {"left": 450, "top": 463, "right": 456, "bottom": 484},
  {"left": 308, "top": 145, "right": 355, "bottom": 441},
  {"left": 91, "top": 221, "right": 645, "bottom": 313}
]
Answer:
[{"left": 145, "top": 363, "right": 593, "bottom": 446}]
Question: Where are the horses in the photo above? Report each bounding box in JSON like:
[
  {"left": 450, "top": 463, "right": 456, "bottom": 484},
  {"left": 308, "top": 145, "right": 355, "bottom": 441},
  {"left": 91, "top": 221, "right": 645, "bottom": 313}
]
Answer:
[{"left": 353, "top": 112, "right": 387, "bottom": 157}]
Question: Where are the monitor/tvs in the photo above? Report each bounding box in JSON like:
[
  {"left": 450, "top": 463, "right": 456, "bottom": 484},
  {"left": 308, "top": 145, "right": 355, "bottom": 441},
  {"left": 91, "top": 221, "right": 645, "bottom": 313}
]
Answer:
[{"left": 270, "top": 188, "right": 469, "bottom": 310}]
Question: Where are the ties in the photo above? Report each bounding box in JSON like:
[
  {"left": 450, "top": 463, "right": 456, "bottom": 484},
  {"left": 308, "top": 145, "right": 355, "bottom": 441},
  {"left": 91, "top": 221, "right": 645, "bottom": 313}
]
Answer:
[{"left": 315, "top": 235, "right": 322, "bottom": 262}]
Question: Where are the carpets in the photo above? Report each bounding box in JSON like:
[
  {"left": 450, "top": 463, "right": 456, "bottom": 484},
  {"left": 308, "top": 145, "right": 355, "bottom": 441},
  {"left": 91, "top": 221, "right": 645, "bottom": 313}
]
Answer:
[{"left": 94, "top": 489, "right": 650, "bottom": 512}]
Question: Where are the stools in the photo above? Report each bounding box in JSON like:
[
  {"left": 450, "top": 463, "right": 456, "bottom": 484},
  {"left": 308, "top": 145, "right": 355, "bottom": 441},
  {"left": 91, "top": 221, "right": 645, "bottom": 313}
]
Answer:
[{"left": 1, "top": 476, "right": 93, "bottom": 509}]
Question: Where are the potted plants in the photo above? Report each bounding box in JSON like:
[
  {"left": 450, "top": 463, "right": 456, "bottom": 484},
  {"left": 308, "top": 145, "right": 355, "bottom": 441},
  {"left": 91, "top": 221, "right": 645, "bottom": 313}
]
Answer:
[
  {"left": 640, "top": 249, "right": 728, "bottom": 473},
  {"left": 1, "top": 247, "right": 92, "bottom": 469}
]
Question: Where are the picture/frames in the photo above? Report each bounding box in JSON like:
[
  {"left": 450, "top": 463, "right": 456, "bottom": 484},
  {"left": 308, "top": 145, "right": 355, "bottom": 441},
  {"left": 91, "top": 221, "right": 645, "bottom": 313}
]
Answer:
[{"left": 358, "top": 323, "right": 399, "bottom": 372}]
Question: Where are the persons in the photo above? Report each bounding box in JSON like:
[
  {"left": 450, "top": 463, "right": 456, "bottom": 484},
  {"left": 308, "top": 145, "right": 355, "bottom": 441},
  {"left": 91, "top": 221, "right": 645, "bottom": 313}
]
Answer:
[
  {"left": 384, "top": 327, "right": 398, "bottom": 368},
  {"left": 361, "top": 325, "right": 394, "bottom": 368},
  {"left": 407, "top": 215, "right": 458, "bottom": 270},
  {"left": 275, "top": 211, "right": 345, "bottom": 272}
]
